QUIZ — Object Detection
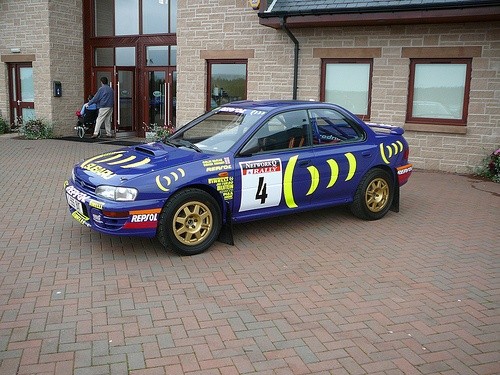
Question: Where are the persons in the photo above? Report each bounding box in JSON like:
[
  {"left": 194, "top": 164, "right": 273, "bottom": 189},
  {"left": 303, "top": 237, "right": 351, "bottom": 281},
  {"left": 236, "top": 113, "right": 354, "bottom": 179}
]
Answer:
[
  {"left": 77, "top": 94, "right": 96, "bottom": 127},
  {"left": 88, "top": 76, "right": 114, "bottom": 139}
]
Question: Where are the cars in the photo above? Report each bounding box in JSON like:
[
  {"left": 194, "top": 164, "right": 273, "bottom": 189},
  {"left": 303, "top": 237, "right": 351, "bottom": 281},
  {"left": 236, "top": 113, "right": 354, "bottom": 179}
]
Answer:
[{"left": 63, "top": 100, "right": 412, "bottom": 256}]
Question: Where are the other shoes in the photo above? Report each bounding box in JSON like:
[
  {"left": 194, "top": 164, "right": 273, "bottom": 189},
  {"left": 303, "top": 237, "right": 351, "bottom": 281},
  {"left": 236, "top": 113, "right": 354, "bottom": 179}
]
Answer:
[
  {"left": 106, "top": 134, "right": 113, "bottom": 138},
  {"left": 91, "top": 135, "right": 97, "bottom": 138}
]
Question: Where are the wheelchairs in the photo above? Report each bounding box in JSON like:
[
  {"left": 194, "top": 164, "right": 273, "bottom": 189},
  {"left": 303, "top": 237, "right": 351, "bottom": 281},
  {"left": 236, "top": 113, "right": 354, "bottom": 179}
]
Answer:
[{"left": 73, "top": 106, "right": 102, "bottom": 139}]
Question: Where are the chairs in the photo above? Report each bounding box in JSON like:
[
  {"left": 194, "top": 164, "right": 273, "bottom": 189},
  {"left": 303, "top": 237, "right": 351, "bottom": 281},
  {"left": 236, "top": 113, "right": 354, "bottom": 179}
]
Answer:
[{"left": 211, "top": 116, "right": 307, "bottom": 159}]
